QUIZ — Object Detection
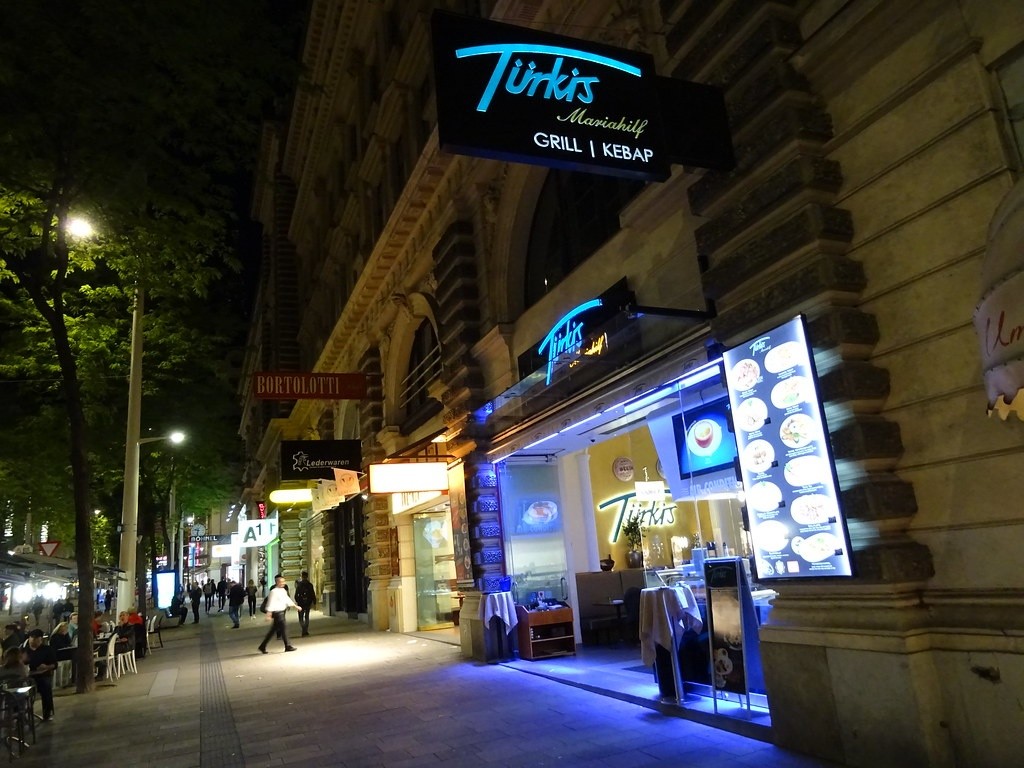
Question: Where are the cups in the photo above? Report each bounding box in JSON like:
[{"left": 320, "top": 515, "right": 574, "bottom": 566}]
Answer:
[{"left": 694, "top": 421, "right": 713, "bottom": 448}]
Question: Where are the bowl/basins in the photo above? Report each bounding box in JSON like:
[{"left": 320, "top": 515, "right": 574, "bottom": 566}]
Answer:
[{"left": 600, "top": 559, "right": 615, "bottom": 571}]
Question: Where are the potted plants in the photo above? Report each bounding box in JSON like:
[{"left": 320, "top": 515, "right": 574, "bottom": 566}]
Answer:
[{"left": 622, "top": 516, "right": 647, "bottom": 569}]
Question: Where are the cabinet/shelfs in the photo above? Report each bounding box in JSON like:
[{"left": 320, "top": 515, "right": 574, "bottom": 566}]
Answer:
[{"left": 515, "top": 599, "right": 577, "bottom": 661}]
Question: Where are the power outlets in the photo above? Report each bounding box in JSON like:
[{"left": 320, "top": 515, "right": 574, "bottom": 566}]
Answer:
[{"left": 539, "top": 591, "right": 544, "bottom": 600}]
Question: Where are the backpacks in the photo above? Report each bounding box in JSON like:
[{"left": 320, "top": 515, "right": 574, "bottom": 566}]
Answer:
[{"left": 299, "top": 588, "right": 312, "bottom": 609}]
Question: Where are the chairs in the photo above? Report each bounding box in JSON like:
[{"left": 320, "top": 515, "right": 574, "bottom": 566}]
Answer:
[
  {"left": 0, "top": 612, "right": 165, "bottom": 764},
  {"left": 612, "top": 586, "right": 646, "bottom": 646}
]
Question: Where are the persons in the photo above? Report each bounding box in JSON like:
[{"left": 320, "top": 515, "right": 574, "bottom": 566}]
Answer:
[
  {"left": 257, "top": 575, "right": 303, "bottom": 654},
  {"left": 294, "top": 570, "right": 318, "bottom": 637},
  {"left": 187, "top": 580, "right": 203, "bottom": 625},
  {"left": 200, "top": 576, "right": 258, "bottom": 629},
  {"left": 170, "top": 592, "right": 189, "bottom": 628},
  {"left": 0, "top": 587, "right": 144, "bottom": 722}
]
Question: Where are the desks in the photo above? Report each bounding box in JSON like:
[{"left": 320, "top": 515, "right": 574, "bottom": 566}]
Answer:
[
  {"left": 592, "top": 600, "right": 624, "bottom": 640},
  {"left": 451, "top": 596, "right": 466, "bottom": 608}
]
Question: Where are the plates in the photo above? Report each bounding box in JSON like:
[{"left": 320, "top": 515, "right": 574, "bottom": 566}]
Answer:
[
  {"left": 734, "top": 359, "right": 760, "bottom": 391},
  {"left": 770, "top": 376, "right": 805, "bottom": 408},
  {"left": 780, "top": 413, "right": 814, "bottom": 448},
  {"left": 764, "top": 341, "right": 801, "bottom": 374},
  {"left": 688, "top": 418, "right": 722, "bottom": 457},
  {"left": 800, "top": 532, "right": 842, "bottom": 562},
  {"left": 736, "top": 397, "right": 768, "bottom": 432},
  {"left": 751, "top": 481, "right": 782, "bottom": 513},
  {"left": 790, "top": 494, "right": 835, "bottom": 524},
  {"left": 783, "top": 455, "right": 824, "bottom": 487},
  {"left": 746, "top": 439, "right": 775, "bottom": 473},
  {"left": 757, "top": 519, "right": 789, "bottom": 551}
]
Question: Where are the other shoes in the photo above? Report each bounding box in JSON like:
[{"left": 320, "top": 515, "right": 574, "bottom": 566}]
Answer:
[
  {"left": 250, "top": 615, "right": 256, "bottom": 620},
  {"left": 285, "top": 646, "right": 296, "bottom": 652},
  {"left": 231, "top": 625, "right": 240, "bottom": 629},
  {"left": 258, "top": 646, "right": 269, "bottom": 655},
  {"left": 301, "top": 630, "right": 309, "bottom": 637}
]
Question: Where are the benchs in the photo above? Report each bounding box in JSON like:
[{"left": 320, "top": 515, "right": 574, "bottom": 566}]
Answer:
[
  {"left": 165, "top": 606, "right": 181, "bottom": 626},
  {"left": 574, "top": 568, "right": 644, "bottom": 645}
]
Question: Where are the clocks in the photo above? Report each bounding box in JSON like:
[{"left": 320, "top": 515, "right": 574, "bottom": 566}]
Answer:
[{"left": 191, "top": 524, "right": 205, "bottom": 538}]
